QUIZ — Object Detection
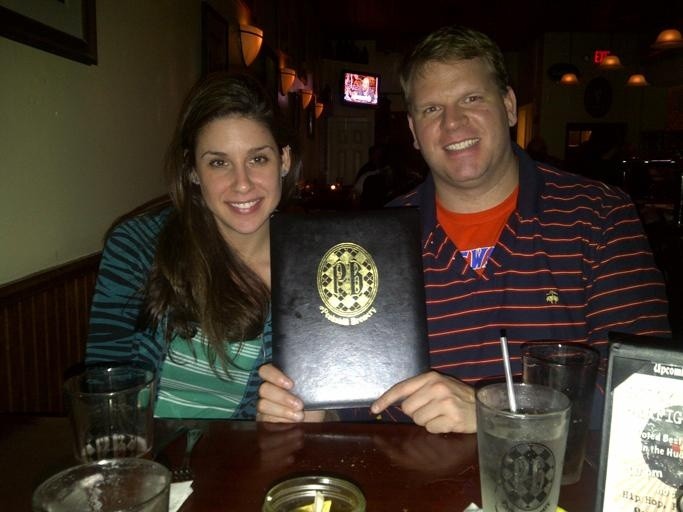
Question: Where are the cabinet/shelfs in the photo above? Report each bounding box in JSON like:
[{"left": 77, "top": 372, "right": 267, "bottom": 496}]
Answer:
[
  {"left": 565, "top": 120, "right": 621, "bottom": 171},
  {"left": 326, "top": 108, "right": 376, "bottom": 186}
]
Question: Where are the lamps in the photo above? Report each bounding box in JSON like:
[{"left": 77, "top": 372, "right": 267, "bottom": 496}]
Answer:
[
  {"left": 650, "top": 29, "right": 683, "bottom": 49},
  {"left": 240, "top": 24, "right": 264, "bottom": 66},
  {"left": 281, "top": 68, "right": 295, "bottom": 96},
  {"left": 315, "top": 104, "right": 323, "bottom": 119},
  {"left": 559, "top": 72, "right": 577, "bottom": 86},
  {"left": 301, "top": 89, "right": 313, "bottom": 108},
  {"left": 598, "top": 55, "right": 624, "bottom": 70},
  {"left": 624, "top": 73, "right": 650, "bottom": 88}
]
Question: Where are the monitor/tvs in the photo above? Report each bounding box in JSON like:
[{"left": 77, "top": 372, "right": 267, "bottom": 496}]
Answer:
[{"left": 343, "top": 69, "right": 380, "bottom": 108}]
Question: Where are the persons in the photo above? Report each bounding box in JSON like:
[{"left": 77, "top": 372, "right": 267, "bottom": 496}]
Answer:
[
  {"left": 354, "top": 143, "right": 426, "bottom": 207},
  {"left": 355, "top": 76, "right": 375, "bottom": 99},
  {"left": 525, "top": 132, "right": 558, "bottom": 165},
  {"left": 255, "top": 26, "right": 677, "bottom": 437},
  {"left": 84, "top": 69, "right": 294, "bottom": 421}
]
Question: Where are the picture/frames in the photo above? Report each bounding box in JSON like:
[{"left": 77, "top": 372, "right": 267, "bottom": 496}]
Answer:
[{"left": 0, "top": 0, "right": 98, "bottom": 66}]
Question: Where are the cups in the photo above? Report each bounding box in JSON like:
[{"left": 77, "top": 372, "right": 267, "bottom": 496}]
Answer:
[
  {"left": 262, "top": 477, "right": 368, "bottom": 512},
  {"left": 475, "top": 381, "right": 573, "bottom": 512},
  {"left": 520, "top": 342, "right": 600, "bottom": 487},
  {"left": 63, "top": 361, "right": 155, "bottom": 462},
  {"left": 31, "top": 457, "right": 173, "bottom": 512}
]
